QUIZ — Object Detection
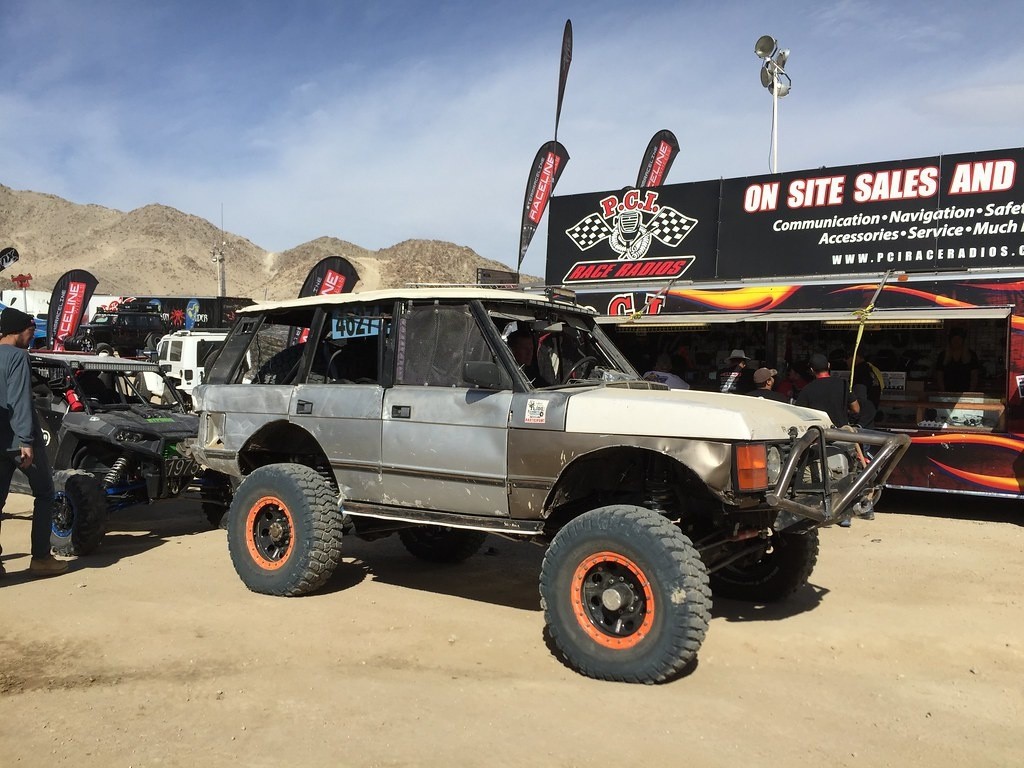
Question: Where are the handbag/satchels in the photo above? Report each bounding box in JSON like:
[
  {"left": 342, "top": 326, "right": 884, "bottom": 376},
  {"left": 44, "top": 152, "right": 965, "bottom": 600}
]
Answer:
[
  {"left": 643, "top": 371, "right": 690, "bottom": 390},
  {"left": 867, "top": 361, "right": 884, "bottom": 394}
]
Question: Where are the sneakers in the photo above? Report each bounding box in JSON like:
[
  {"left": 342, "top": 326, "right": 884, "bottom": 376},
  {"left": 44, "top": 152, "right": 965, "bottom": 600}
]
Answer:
[
  {"left": 30, "top": 555, "right": 68, "bottom": 574},
  {"left": 0, "top": 566, "right": 6, "bottom": 576}
]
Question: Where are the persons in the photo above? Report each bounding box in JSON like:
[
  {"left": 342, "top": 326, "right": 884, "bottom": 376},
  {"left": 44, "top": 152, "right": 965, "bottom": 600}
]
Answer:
[
  {"left": 655, "top": 348, "right": 694, "bottom": 389},
  {"left": 507, "top": 329, "right": 550, "bottom": 389},
  {"left": 935, "top": 327, "right": 979, "bottom": 392},
  {"left": 716, "top": 349, "right": 884, "bottom": 526},
  {"left": 0, "top": 307, "right": 68, "bottom": 580}
]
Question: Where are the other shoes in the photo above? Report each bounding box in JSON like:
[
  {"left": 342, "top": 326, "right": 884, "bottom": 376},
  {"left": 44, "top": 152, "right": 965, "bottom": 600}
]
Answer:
[
  {"left": 836, "top": 518, "right": 851, "bottom": 526},
  {"left": 858, "top": 512, "right": 875, "bottom": 520}
]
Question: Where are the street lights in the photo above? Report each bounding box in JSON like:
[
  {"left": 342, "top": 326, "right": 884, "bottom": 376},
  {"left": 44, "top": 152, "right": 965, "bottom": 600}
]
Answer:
[
  {"left": 752, "top": 33, "right": 791, "bottom": 175},
  {"left": 208, "top": 241, "right": 225, "bottom": 297}
]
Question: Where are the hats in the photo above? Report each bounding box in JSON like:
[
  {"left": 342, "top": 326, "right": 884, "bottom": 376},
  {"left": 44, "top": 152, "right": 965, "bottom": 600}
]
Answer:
[
  {"left": 753, "top": 368, "right": 778, "bottom": 383},
  {"left": 728, "top": 350, "right": 751, "bottom": 361},
  {"left": 807, "top": 354, "right": 829, "bottom": 370},
  {"left": 948, "top": 326, "right": 966, "bottom": 341},
  {"left": 0, "top": 308, "right": 36, "bottom": 336},
  {"left": 792, "top": 361, "right": 808, "bottom": 375}
]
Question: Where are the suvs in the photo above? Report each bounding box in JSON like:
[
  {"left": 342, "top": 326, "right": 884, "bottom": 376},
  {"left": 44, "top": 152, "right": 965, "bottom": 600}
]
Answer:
[
  {"left": 192, "top": 285, "right": 912, "bottom": 685},
  {"left": 1, "top": 300, "right": 255, "bottom": 557}
]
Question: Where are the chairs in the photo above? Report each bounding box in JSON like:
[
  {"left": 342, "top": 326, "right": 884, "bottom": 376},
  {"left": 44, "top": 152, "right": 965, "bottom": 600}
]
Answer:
[{"left": 72, "top": 369, "right": 114, "bottom": 404}]
